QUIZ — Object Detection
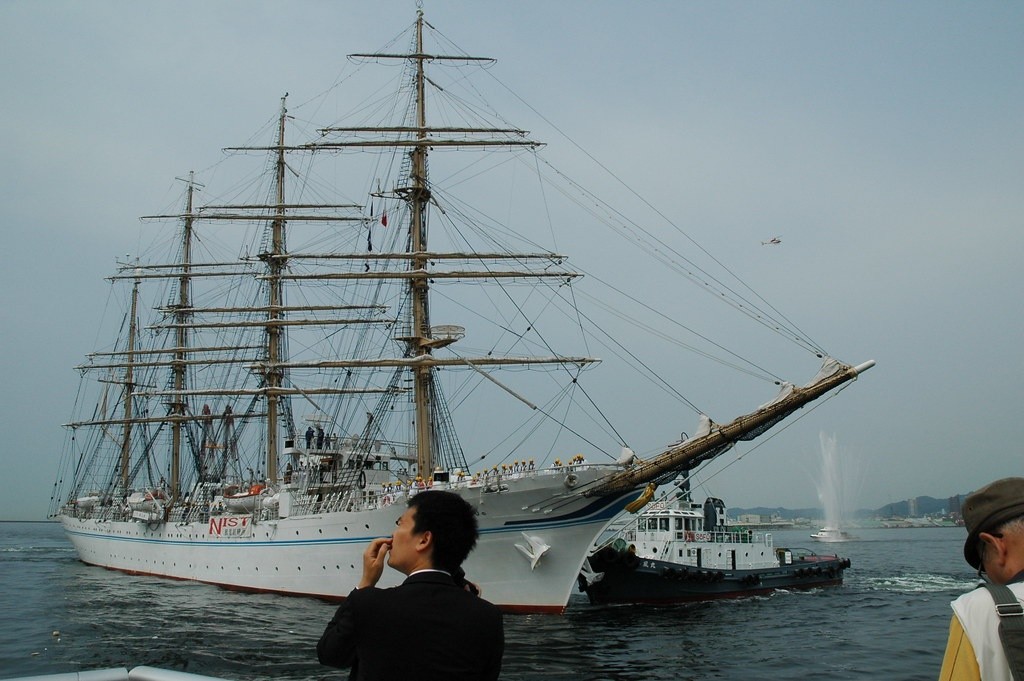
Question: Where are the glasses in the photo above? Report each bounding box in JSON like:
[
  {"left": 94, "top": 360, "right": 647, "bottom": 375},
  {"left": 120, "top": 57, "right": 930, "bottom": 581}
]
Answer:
[{"left": 977, "top": 532, "right": 1004, "bottom": 583}]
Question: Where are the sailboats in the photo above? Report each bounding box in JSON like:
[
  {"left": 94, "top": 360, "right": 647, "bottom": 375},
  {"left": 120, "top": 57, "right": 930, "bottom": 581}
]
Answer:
[{"left": 45, "top": 0, "right": 876, "bottom": 610}]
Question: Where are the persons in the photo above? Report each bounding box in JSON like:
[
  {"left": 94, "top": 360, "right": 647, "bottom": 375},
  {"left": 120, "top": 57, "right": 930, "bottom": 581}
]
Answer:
[
  {"left": 305, "top": 427, "right": 315, "bottom": 448},
  {"left": 284, "top": 460, "right": 331, "bottom": 485},
  {"left": 331, "top": 433, "right": 338, "bottom": 449},
  {"left": 938, "top": 477, "right": 1023, "bottom": 681},
  {"left": 382, "top": 454, "right": 592, "bottom": 493},
  {"left": 325, "top": 433, "right": 331, "bottom": 450},
  {"left": 317, "top": 427, "right": 324, "bottom": 449},
  {"left": 318, "top": 491, "right": 505, "bottom": 678}
]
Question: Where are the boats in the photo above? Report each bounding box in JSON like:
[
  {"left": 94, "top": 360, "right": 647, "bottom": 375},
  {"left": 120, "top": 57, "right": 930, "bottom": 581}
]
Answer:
[
  {"left": 577, "top": 434, "right": 852, "bottom": 602},
  {"left": 810, "top": 527, "right": 860, "bottom": 542}
]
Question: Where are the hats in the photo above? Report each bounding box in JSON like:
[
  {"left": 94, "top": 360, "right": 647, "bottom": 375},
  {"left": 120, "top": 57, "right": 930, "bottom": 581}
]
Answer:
[{"left": 962, "top": 477, "right": 1024, "bottom": 573}]
